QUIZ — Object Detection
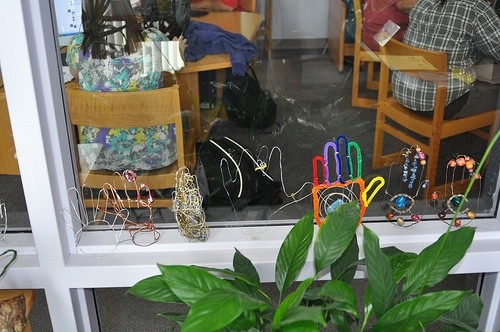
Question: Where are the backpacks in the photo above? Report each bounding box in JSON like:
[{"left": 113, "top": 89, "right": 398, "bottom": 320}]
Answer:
[
  {"left": 223, "top": 64, "right": 280, "bottom": 134},
  {"left": 201, "top": 136, "right": 284, "bottom": 211}
]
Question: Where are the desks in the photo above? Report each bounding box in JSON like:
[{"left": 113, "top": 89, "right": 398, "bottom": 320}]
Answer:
[
  {"left": 71, "top": 8, "right": 263, "bottom": 143},
  {"left": 0, "top": 289, "right": 36, "bottom": 332}
]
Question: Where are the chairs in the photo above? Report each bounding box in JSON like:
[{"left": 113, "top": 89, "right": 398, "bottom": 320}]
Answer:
[
  {"left": 239, "top": 0, "right": 255, "bottom": 77},
  {"left": 372, "top": 31, "right": 500, "bottom": 200},
  {"left": 351, "top": 0, "right": 392, "bottom": 110},
  {"left": 256, "top": 1, "right": 273, "bottom": 64},
  {"left": 64, "top": 83, "right": 198, "bottom": 225},
  {"left": 327, "top": 0, "right": 355, "bottom": 72}
]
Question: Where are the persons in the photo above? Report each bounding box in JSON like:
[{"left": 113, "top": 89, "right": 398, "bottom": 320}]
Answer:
[
  {"left": 188, "top": 0, "right": 238, "bottom": 109},
  {"left": 362, "top": 0, "right": 418, "bottom": 51},
  {"left": 392, "top": 0, "right": 500, "bottom": 141}
]
